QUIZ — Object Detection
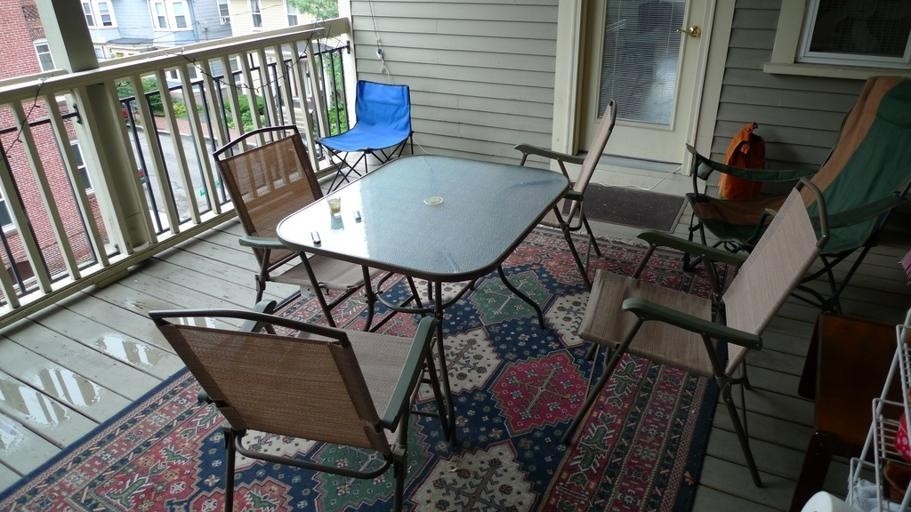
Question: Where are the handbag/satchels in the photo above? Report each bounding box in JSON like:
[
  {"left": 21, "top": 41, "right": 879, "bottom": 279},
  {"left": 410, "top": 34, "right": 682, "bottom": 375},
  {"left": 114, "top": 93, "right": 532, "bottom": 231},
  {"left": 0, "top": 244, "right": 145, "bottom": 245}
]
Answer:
[{"left": 718, "top": 118, "right": 770, "bottom": 203}]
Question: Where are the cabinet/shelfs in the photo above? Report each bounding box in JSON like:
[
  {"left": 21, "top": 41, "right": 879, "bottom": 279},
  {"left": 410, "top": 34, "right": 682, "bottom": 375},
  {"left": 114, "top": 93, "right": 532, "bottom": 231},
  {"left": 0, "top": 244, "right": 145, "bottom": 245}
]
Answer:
[{"left": 845, "top": 307, "right": 911, "bottom": 512}]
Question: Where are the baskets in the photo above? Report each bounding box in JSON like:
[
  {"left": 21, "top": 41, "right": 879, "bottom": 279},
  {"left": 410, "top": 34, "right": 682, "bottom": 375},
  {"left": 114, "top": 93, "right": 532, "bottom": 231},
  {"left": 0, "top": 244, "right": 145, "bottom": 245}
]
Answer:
[{"left": 882, "top": 458, "right": 911, "bottom": 503}]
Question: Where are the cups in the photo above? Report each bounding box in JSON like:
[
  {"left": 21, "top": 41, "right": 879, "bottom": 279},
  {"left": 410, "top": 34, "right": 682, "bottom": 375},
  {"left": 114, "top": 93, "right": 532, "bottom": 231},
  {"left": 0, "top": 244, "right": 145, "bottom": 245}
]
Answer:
[
  {"left": 326, "top": 195, "right": 342, "bottom": 216},
  {"left": 329, "top": 213, "right": 344, "bottom": 231}
]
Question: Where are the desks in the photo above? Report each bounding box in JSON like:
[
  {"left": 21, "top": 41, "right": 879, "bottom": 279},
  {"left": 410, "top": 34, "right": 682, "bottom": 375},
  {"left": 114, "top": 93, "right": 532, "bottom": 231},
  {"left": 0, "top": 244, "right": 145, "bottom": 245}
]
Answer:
[{"left": 787, "top": 313, "right": 911, "bottom": 512}]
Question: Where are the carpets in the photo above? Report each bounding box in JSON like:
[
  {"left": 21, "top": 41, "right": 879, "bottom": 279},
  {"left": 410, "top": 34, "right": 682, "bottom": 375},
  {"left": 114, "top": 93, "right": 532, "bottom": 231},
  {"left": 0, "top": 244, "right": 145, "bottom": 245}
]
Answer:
[{"left": 0, "top": 225, "right": 742, "bottom": 512}]
